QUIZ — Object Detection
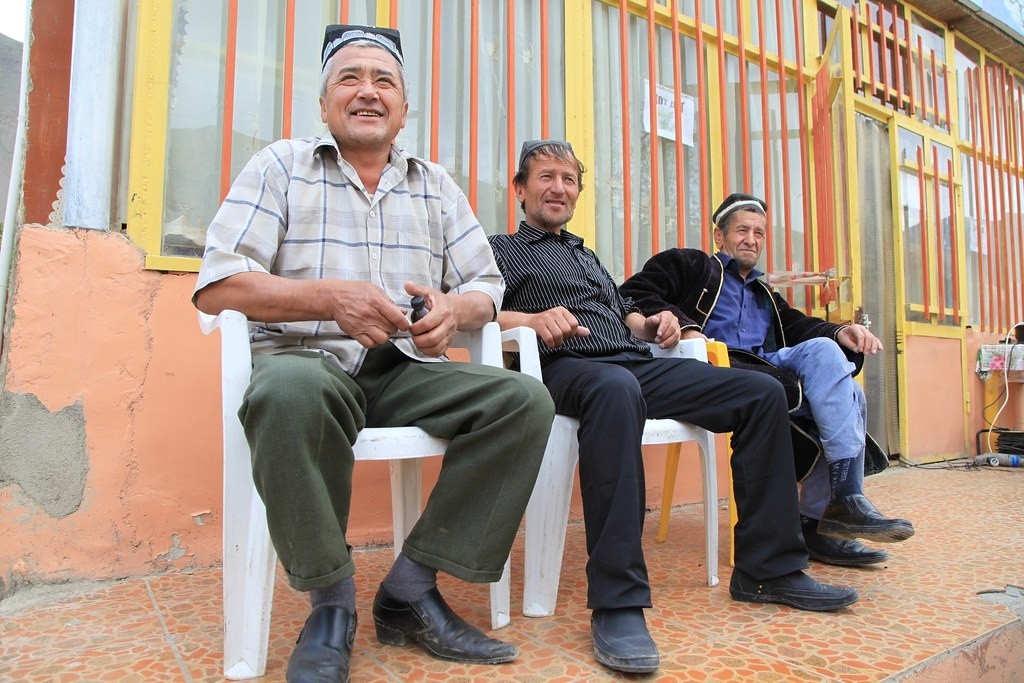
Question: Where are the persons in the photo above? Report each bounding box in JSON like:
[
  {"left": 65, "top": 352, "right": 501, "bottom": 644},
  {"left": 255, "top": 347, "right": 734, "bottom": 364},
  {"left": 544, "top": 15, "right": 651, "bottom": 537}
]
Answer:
[
  {"left": 189, "top": 24, "right": 556, "bottom": 683},
  {"left": 487, "top": 139, "right": 860, "bottom": 675},
  {"left": 619, "top": 194, "right": 915, "bottom": 566}
]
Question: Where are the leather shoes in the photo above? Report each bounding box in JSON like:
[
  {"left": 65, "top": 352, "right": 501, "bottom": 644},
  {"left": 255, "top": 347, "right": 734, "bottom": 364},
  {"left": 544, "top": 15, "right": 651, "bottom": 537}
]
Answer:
[
  {"left": 816, "top": 494, "right": 916, "bottom": 543},
  {"left": 805, "top": 532, "right": 889, "bottom": 565},
  {"left": 590, "top": 607, "right": 661, "bottom": 672},
  {"left": 372, "top": 580, "right": 518, "bottom": 664},
  {"left": 729, "top": 566, "right": 859, "bottom": 610},
  {"left": 286, "top": 606, "right": 358, "bottom": 683}
]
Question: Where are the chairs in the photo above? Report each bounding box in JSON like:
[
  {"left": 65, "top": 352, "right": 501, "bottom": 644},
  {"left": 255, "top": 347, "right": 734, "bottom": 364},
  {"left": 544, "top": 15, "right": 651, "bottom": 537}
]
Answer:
[
  {"left": 501, "top": 326, "right": 717, "bottom": 618},
  {"left": 654, "top": 342, "right": 739, "bottom": 567},
  {"left": 197, "top": 303, "right": 513, "bottom": 680}
]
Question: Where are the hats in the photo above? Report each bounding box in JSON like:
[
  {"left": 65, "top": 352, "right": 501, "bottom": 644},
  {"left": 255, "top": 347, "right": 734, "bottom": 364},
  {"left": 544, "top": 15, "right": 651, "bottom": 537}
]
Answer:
[
  {"left": 518, "top": 140, "right": 574, "bottom": 171},
  {"left": 320, "top": 24, "right": 405, "bottom": 72},
  {"left": 714, "top": 192, "right": 768, "bottom": 228}
]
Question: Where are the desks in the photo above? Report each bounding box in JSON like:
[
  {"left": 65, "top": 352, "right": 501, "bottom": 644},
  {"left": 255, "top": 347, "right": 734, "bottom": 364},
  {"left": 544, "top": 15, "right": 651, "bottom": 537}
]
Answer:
[{"left": 977, "top": 344, "right": 1024, "bottom": 454}]
